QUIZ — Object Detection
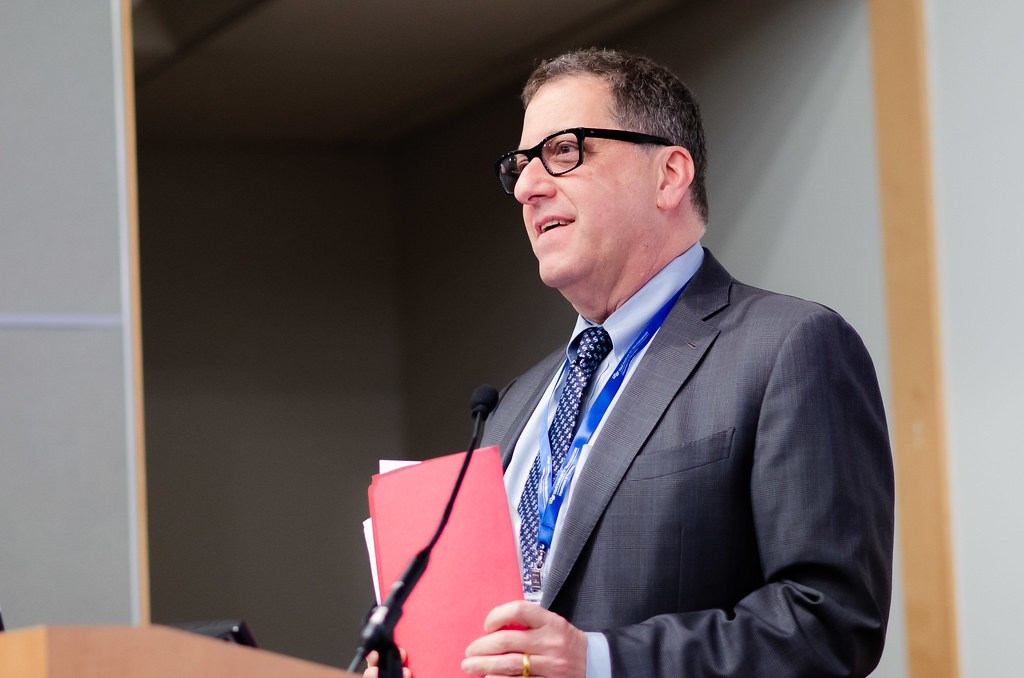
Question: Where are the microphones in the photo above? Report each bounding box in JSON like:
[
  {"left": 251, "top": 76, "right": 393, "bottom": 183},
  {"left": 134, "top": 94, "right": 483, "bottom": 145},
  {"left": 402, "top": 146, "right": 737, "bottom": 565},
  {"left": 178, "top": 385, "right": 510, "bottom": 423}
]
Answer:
[{"left": 348, "top": 384, "right": 500, "bottom": 676}]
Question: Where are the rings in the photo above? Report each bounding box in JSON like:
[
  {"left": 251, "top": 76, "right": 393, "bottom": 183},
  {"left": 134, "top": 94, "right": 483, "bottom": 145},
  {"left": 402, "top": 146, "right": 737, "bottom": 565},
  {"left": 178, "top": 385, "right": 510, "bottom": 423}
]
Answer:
[{"left": 523, "top": 654, "right": 530, "bottom": 676}]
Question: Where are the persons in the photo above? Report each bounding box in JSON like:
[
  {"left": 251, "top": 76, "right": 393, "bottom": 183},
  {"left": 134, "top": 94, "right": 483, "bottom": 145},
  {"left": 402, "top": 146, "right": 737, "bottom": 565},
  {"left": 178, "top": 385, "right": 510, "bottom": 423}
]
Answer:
[{"left": 364, "top": 49, "right": 895, "bottom": 678}]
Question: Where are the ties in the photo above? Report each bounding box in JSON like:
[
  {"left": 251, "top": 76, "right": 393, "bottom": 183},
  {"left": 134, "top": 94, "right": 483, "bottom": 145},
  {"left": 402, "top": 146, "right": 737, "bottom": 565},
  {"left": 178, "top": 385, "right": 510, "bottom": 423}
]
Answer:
[{"left": 515, "top": 325, "right": 613, "bottom": 595}]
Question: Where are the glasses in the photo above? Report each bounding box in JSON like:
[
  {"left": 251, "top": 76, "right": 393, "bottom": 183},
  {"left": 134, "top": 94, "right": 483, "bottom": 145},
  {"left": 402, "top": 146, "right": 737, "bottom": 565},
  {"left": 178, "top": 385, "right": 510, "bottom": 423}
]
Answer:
[{"left": 498, "top": 126, "right": 675, "bottom": 194}]
